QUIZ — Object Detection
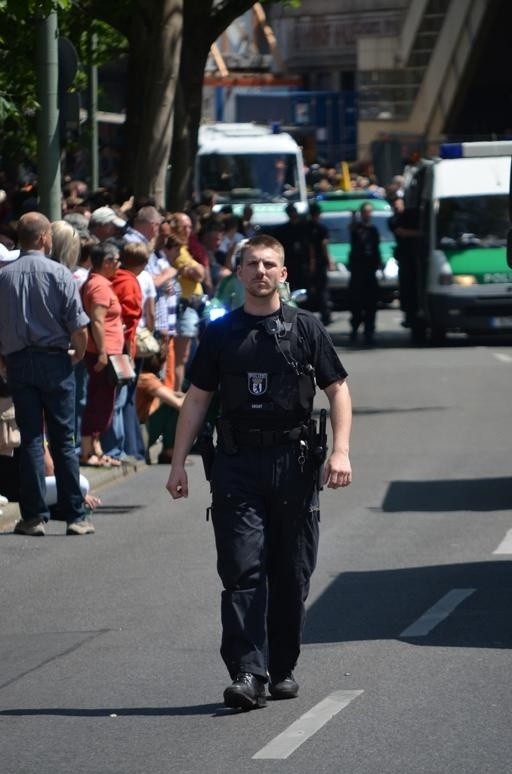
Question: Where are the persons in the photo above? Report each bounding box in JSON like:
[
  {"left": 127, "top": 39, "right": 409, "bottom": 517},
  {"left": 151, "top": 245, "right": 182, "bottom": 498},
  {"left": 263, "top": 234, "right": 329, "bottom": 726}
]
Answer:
[
  {"left": 387, "top": 198, "right": 405, "bottom": 262},
  {"left": 276, "top": 203, "right": 330, "bottom": 344},
  {"left": 166, "top": 234, "right": 352, "bottom": 711},
  {"left": 303, "top": 166, "right": 405, "bottom": 205},
  {"left": 1, "top": 165, "right": 263, "bottom": 535},
  {"left": 347, "top": 202, "right": 385, "bottom": 346}
]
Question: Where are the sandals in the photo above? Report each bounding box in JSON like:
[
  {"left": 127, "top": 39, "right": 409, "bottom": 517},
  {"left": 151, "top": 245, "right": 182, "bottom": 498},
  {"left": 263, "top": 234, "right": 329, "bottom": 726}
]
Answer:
[{"left": 79, "top": 452, "right": 122, "bottom": 468}]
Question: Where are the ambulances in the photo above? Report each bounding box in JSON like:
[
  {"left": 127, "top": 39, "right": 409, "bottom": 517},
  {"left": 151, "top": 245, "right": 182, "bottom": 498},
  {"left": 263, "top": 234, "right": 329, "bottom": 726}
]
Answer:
[
  {"left": 404, "top": 142, "right": 511, "bottom": 342},
  {"left": 196, "top": 121, "right": 310, "bottom": 231},
  {"left": 310, "top": 192, "right": 400, "bottom": 310}
]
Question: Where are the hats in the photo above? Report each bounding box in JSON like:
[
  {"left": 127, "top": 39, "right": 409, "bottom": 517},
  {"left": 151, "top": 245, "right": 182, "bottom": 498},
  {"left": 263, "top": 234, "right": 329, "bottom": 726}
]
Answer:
[{"left": 88, "top": 204, "right": 129, "bottom": 230}]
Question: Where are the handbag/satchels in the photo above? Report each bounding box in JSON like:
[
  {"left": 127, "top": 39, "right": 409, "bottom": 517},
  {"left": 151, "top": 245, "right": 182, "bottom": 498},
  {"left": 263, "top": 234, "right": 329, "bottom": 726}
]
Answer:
[
  {"left": 126, "top": 326, "right": 161, "bottom": 358},
  {"left": 107, "top": 352, "right": 137, "bottom": 383}
]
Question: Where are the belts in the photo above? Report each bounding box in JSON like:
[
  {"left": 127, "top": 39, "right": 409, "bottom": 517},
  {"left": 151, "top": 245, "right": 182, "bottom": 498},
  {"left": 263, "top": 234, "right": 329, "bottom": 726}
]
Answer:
[{"left": 235, "top": 426, "right": 304, "bottom": 445}]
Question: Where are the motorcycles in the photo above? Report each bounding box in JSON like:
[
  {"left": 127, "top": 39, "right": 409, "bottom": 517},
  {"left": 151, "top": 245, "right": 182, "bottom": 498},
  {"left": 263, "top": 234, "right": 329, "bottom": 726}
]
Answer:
[{"left": 193, "top": 272, "right": 309, "bottom": 484}]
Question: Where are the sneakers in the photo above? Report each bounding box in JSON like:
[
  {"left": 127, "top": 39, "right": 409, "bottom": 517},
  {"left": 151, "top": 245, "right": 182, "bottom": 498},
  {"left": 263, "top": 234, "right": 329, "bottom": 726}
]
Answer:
[
  {"left": 65, "top": 520, "right": 96, "bottom": 536},
  {"left": 265, "top": 665, "right": 298, "bottom": 701},
  {"left": 222, "top": 672, "right": 266, "bottom": 710},
  {"left": 14, "top": 518, "right": 46, "bottom": 536},
  {"left": 156, "top": 450, "right": 194, "bottom": 466}
]
